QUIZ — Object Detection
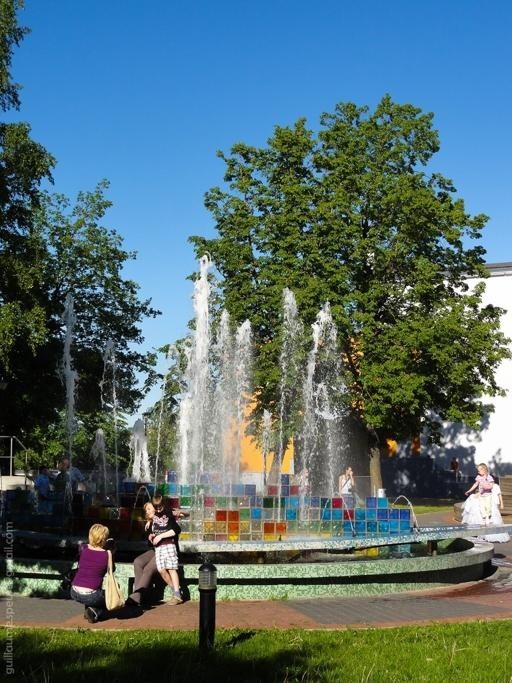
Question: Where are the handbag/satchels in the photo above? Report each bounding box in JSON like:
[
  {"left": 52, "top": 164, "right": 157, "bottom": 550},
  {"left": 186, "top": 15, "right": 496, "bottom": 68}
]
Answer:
[
  {"left": 116, "top": 598, "right": 145, "bottom": 619},
  {"left": 105, "top": 574, "right": 124, "bottom": 610}
]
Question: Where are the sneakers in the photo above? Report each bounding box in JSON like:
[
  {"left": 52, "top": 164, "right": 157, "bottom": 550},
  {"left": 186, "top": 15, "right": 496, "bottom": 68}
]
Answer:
[
  {"left": 166, "top": 597, "right": 183, "bottom": 606},
  {"left": 87, "top": 607, "right": 98, "bottom": 623}
]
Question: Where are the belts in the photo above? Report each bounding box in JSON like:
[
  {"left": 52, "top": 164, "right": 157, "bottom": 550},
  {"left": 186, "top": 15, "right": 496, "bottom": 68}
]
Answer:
[{"left": 72, "top": 586, "right": 97, "bottom": 595}]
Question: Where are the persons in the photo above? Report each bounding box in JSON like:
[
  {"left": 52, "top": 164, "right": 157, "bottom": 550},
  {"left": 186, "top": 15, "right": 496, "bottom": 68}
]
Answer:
[
  {"left": 460, "top": 464, "right": 494, "bottom": 525},
  {"left": 338, "top": 467, "right": 356, "bottom": 494},
  {"left": 70, "top": 522, "right": 109, "bottom": 623},
  {"left": 450, "top": 456, "right": 460, "bottom": 475},
  {"left": 22, "top": 458, "right": 90, "bottom": 514},
  {"left": 132, "top": 492, "right": 184, "bottom": 607},
  {"left": 475, "top": 475, "right": 510, "bottom": 544}
]
